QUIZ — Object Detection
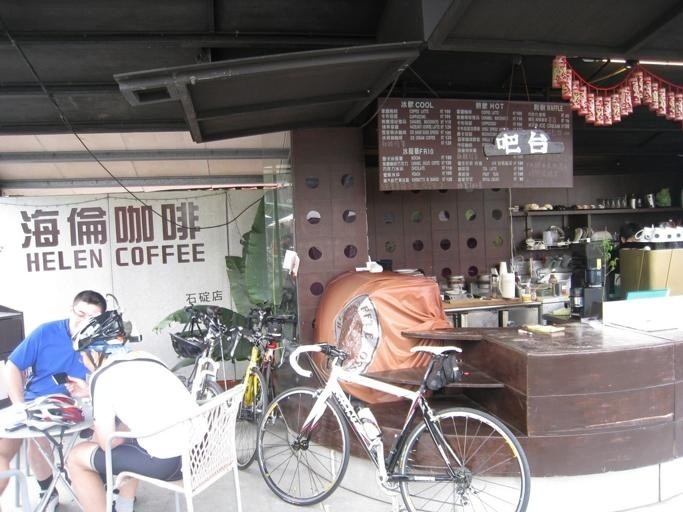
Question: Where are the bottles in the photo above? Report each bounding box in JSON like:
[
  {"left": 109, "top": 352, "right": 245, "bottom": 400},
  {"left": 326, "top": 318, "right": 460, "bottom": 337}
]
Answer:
[{"left": 356, "top": 404, "right": 383, "bottom": 442}]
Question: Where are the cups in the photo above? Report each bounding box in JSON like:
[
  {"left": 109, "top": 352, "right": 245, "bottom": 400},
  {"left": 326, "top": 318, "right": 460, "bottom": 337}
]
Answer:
[
  {"left": 543, "top": 231, "right": 553, "bottom": 245},
  {"left": 498, "top": 273, "right": 516, "bottom": 299}
]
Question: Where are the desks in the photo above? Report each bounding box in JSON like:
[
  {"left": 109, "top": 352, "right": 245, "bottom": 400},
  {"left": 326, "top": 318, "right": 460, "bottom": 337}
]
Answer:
[{"left": 0, "top": 403, "right": 95, "bottom": 511}]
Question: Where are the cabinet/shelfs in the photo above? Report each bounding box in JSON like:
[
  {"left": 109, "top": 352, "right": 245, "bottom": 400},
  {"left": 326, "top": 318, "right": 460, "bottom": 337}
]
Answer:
[{"left": 443, "top": 203, "right": 683, "bottom": 325}]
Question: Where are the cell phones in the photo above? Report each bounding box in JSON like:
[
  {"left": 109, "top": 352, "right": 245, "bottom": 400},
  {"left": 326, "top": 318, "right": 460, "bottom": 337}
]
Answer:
[
  {"left": 5, "top": 423, "right": 26, "bottom": 431},
  {"left": 52, "top": 372, "right": 69, "bottom": 385}
]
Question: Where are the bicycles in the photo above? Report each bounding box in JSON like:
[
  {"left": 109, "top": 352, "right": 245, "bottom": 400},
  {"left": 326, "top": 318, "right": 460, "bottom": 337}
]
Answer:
[
  {"left": 160, "top": 301, "right": 234, "bottom": 448},
  {"left": 256, "top": 332, "right": 534, "bottom": 512},
  {"left": 224, "top": 301, "right": 298, "bottom": 470}
]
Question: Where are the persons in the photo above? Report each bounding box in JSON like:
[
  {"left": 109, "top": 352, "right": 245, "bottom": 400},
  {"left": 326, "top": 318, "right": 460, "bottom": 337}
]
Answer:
[
  {"left": 62, "top": 310, "right": 214, "bottom": 511},
  {"left": 0, "top": 288, "right": 106, "bottom": 511}
]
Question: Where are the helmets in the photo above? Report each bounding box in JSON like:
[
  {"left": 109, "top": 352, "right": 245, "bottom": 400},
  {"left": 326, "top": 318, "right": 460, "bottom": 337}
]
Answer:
[
  {"left": 25, "top": 393, "right": 84, "bottom": 429},
  {"left": 171, "top": 334, "right": 209, "bottom": 357},
  {"left": 72, "top": 310, "right": 125, "bottom": 351}
]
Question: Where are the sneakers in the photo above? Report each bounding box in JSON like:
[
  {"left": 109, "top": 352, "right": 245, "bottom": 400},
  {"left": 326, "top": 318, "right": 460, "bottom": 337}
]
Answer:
[{"left": 40, "top": 489, "right": 59, "bottom": 512}]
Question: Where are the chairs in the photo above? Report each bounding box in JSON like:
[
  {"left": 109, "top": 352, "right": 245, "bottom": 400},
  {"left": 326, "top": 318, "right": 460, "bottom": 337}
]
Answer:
[{"left": 106, "top": 383, "right": 246, "bottom": 512}]
